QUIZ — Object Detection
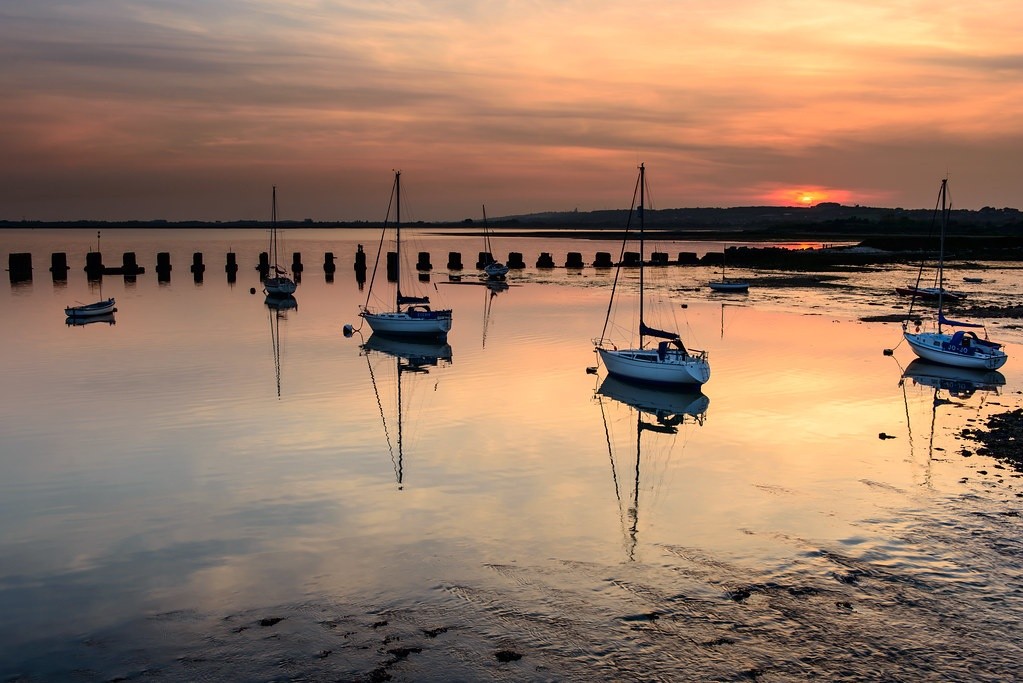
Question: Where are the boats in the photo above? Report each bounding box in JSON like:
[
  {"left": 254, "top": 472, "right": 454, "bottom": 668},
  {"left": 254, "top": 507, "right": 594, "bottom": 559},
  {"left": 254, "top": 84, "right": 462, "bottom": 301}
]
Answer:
[
  {"left": 65, "top": 298, "right": 118, "bottom": 317},
  {"left": 65, "top": 312, "right": 116, "bottom": 328}
]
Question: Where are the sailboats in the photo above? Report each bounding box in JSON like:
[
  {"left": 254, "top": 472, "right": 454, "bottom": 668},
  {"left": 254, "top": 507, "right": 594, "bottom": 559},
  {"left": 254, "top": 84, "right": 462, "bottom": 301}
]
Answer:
[
  {"left": 585, "top": 160, "right": 711, "bottom": 394},
  {"left": 893, "top": 177, "right": 1009, "bottom": 374},
  {"left": 896, "top": 358, "right": 1008, "bottom": 464},
  {"left": 590, "top": 374, "right": 712, "bottom": 564},
  {"left": 351, "top": 167, "right": 453, "bottom": 336},
  {"left": 260, "top": 184, "right": 298, "bottom": 294},
  {"left": 357, "top": 332, "right": 456, "bottom": 492},
  {"left": 481, "top": 280, "right": 510, "bottom": 349},
  {"left": 481, "top": 203, "right": 509, "bottom": 277},
  {"left": 264, "top": 295, "right": 299, "bottom": 401},
  {"left": 708, "top": 243, "right": 750, "bottom": 291}
]
